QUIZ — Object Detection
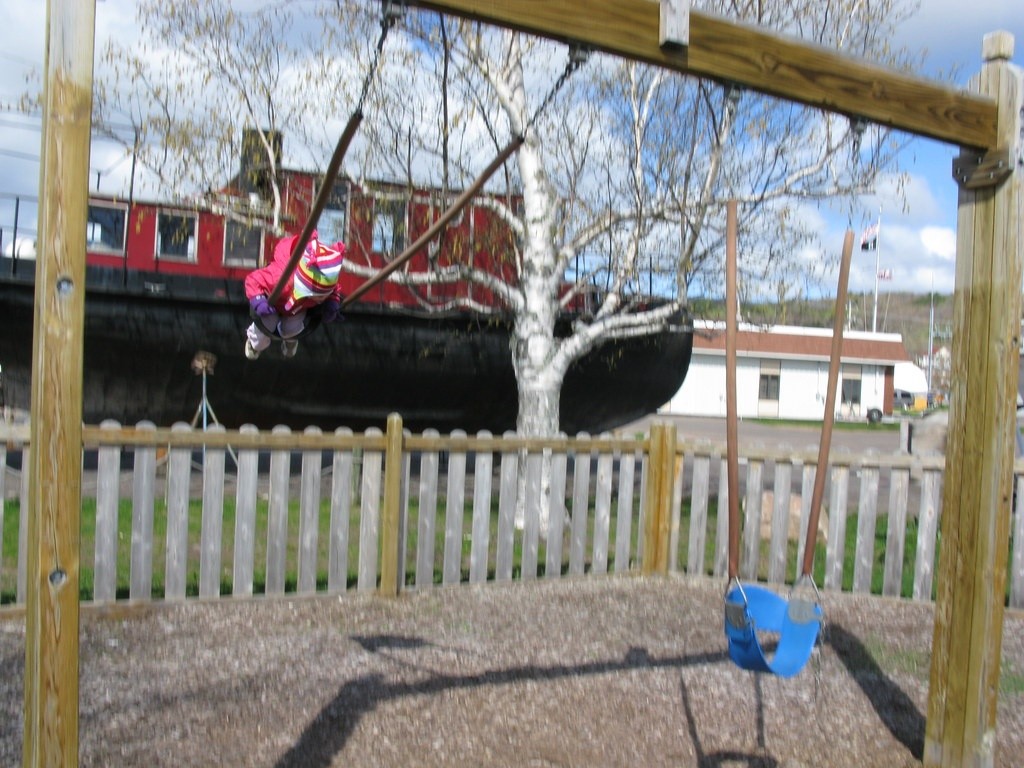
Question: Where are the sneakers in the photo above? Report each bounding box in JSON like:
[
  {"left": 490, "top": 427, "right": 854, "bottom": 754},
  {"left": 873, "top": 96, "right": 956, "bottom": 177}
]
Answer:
[
  {"left": 281, "top": 337, "right": 299, "bottom": 357},
  {"left": 245, "top": 339, "right": 259, "bottom": 359}
]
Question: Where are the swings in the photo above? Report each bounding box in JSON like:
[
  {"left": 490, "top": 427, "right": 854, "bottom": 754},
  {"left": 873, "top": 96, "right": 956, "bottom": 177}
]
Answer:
[
  {"left": 249, "top": 19, "right": 595, "bottom": 345},
  {"left": 719, "top": 84, "right": 867, "bottom": 678}
]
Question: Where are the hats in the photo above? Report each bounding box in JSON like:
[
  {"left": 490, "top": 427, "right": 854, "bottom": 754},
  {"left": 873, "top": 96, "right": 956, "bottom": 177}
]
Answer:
[{"left": 285, "top": 244, "right": 343, "bottom": 311}]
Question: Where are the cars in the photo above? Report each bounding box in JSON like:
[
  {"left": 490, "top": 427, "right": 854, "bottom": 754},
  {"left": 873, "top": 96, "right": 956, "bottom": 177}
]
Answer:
[{"left": 893, "top": 388, "right": 915, "bottom": 413}]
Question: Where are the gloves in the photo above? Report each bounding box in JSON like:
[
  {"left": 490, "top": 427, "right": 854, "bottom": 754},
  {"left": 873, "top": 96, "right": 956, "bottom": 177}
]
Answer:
[
  {"left": 319, "top": 300, "right": 344, "bottom": 323},
  {"left": 249, "top": 295, "right": 274, "bottom": 317}
]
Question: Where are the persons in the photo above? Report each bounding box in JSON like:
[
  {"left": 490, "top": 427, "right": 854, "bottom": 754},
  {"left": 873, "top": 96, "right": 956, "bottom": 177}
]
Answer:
[{"left": 244, "top": 229, "right": 346, "bottom": 360}]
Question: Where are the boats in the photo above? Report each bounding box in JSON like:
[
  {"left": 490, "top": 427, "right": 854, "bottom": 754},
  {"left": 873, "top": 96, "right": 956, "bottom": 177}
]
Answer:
[{"left": 1, "top": 128, "right": 697, "bottom": 439}]
